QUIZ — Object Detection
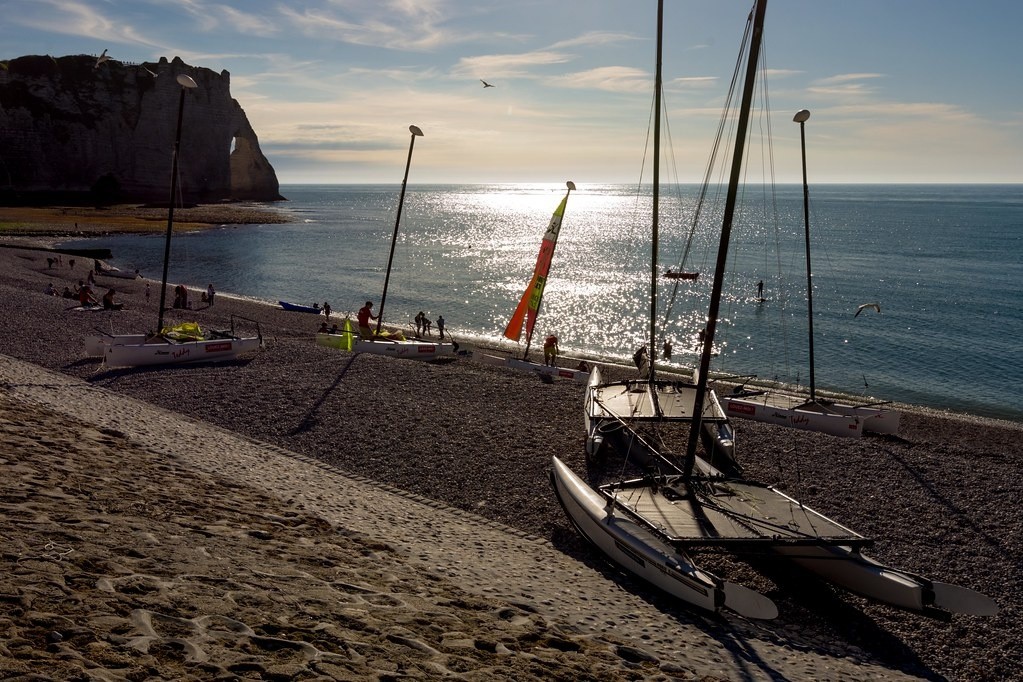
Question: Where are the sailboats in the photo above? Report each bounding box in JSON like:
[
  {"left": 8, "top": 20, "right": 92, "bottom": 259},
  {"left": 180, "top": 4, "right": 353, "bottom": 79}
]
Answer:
[
  {"left": 469, "top": 179, "right": 590, "bottom": 384},
  {"left": 720, "top": 108, "right": 903, "bottom": 440},
  {"left": 547, "top": 1, "right": 1002, "bottom": 623},
  {"left": 314, "top": 123, "right": 463, "bottom": 360},
  {"left": 83, "top": 74, "right": 266, "bottom": 376}
]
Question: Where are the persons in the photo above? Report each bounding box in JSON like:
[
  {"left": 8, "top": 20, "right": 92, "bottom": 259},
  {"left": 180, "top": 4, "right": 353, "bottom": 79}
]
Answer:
[
  {"left": 318, "top": 322, "right": 338, "bottom": 334},
  {"left": 175, "top": 286, "right": 187, "bottom": 308},
  {"left": 47, "top": 255, "right": 62, "bottom": 268},
  {"left": 74, "top": 270, "right": 98, "bottom": 306},
  {"left": 145, "top": 283, "right": 151, "bottom": 300},
  {"left": 662, "top": 339, "right": 672, "bottom": 360},
  {"left": 208, "top": 284, "right": 214, "bottom": 307},
  {"left": 69, "top": 259, "right": 75, "bottom": 269},
  {"left": 757, "top": 280, "right": 763, "bottom": 298},
  {"left": 94, "top": 259, "right": 101, "bottom": 275},
  {"left": 48, "top": 283, "right": 73, "bottom": 299},
  {"left": 357, "top": 301, "right": 379, "bottom": 335},
  {"left": 416, "top": 311, "right": 429, "bottom": 336},
  {"left": 103, "top": 289, "right": 123, "bottom": 311},
  {"left": 437, "top": 316, "right": 444, "bottom": 340},
  {"left": 324, "top": 302, "right": 330, "bottom": 320},
  {"left": 544, "top": 334, "right": 559, "bottom": 367},
  {"left": 201, "top": 292, "right": 207, "bottom": 301}
]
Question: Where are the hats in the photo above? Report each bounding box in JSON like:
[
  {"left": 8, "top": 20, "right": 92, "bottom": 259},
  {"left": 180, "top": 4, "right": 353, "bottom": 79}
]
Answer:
[
  {"left": 333, "top": 325, "right": 338, "bottom": 328},
  {"left": 111, "top": 289, "right": 115, "bottom": 295},
  {"left": 321, "top": 323, "right": 327, "bottom": 326}
]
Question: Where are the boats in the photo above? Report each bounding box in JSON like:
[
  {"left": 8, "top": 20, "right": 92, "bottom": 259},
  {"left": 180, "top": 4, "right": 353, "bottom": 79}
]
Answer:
[
  {"left": 278, "top": 300, "right": 326, "bottom": 314},
  {"left": 663, "top": 269, "right": 700, "bottom": 280}
]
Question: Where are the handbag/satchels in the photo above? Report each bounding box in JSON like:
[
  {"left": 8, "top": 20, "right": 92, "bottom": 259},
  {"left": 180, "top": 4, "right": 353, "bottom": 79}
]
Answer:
[{"left": 213, "top": 292, "right": 215, "bottom": 294}]
